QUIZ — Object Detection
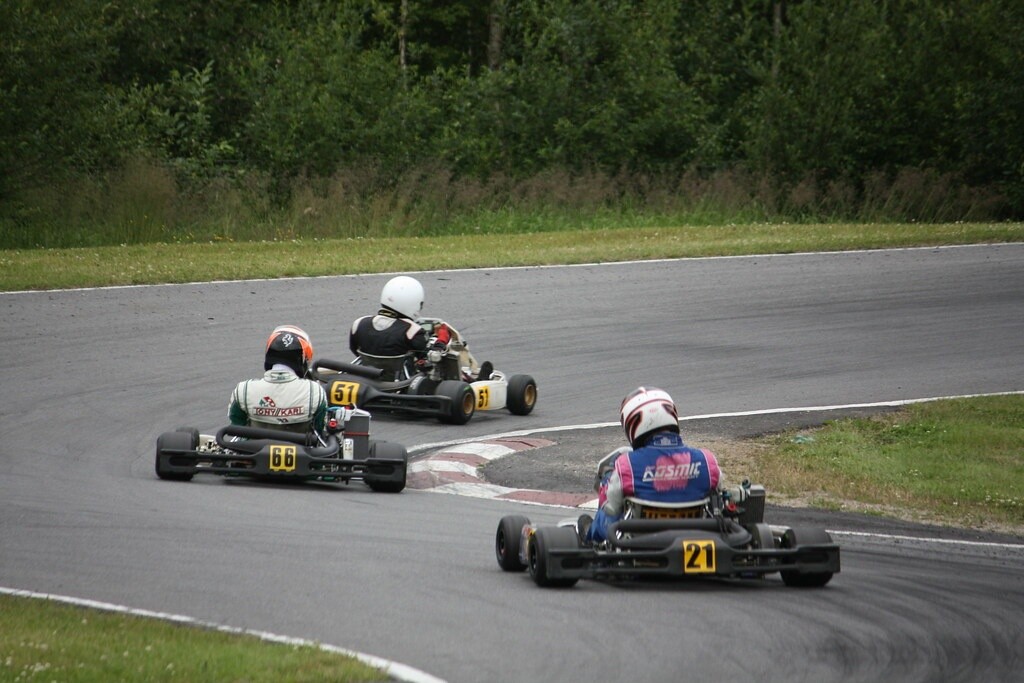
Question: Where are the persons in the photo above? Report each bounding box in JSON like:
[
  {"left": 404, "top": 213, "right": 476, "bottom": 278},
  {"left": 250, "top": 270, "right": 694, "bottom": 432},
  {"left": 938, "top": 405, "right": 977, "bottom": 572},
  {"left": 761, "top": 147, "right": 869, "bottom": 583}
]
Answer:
[
  {"left": 578, "top": 386, "right": 723, "bottom": 547},
  {"left": 229, "top": 324, "right": 328, "bottom": 434},
  {"left": 349, "top": 276, "right": 493, "bottom": 382}
]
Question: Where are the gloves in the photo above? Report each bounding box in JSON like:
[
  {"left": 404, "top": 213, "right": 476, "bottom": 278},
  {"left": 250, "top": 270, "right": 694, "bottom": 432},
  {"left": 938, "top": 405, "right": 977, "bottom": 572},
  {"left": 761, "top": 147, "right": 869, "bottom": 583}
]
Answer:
[{"left": 434, "top": 324, "right": 453, "bottom": 344}]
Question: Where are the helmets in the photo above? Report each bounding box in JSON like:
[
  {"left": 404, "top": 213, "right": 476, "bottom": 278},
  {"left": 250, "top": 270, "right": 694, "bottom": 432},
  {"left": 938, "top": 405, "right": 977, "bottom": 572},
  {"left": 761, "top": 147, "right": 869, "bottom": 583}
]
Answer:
[
  {"left": 380, "top": 276, "right": 425, "bottom": 321},
  {"left": 263, "top": 324, "right": 314, "bottom": 378},
  {"left": 619, "top": 386, "right": 680, "bottom": 449}
]
